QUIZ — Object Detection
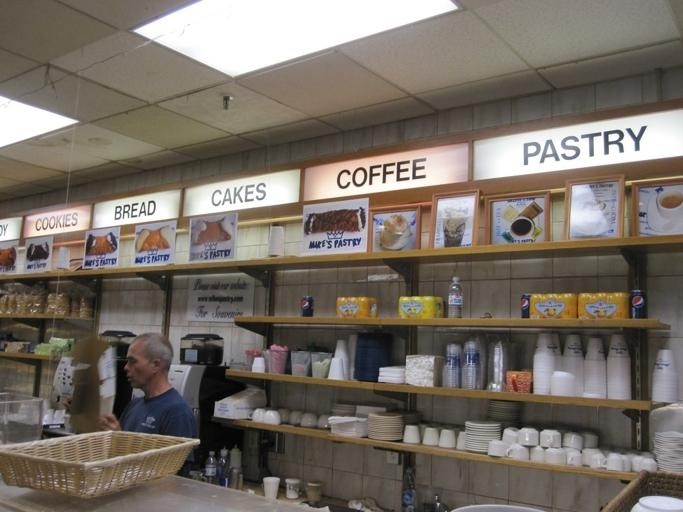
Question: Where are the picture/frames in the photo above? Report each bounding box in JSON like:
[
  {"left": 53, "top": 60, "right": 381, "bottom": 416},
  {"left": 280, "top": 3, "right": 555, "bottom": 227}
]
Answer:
[
  {"left": 561, "top": 173, "right": 626, "bottom": 239},
  {"left": 427, "top": 188, "right": 480, "bottom": 247},
  {"left": 484, "top": 190, "right": 551, "bottom": 245},
  {"left": 367, "top": 202, "right": 420, "bottom": 252},
  {"left": 630, "top": 177, "right": 682, "bottom": 239}
]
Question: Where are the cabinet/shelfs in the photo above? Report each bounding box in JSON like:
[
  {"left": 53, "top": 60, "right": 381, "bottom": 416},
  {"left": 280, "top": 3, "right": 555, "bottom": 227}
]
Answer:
[
  {"left": 208, "top": 314, "right": 670, "bottom": 485},
  {"left": 0, "top": 313, "right": 93, "bottom": 363}
]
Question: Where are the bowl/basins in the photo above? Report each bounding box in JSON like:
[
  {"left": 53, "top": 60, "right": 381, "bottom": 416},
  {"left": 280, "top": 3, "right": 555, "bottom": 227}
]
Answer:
[
  {"left": 448, "top": 503, "right": 544, "bottom": 512},
  {"left": 631, "top": 495, "right": 682, "bottom": 512}
]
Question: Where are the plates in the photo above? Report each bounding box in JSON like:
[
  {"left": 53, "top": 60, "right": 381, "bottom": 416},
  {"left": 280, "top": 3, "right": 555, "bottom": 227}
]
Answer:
[
  {"left": 379, "top": 228, "right": 409, "bottom": 250},
  {"left": 329, "top": 403, "right": 355, "bottom": 417},
  {"left": 464, "top": 400, "right": 521, "bottom": 454},
  {"left": 652, "top": 430, "right": 682, "bottom": 472},
  {"left": 646, "top": 192, "right": 683, "bottom": 232},
  {"left": 366, "top": 411, "right": 417, "bottom": 440}
]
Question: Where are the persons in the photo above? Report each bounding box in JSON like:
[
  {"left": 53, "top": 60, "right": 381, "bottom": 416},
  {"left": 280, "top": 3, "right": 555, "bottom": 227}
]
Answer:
[{"left": 96, "top": 331, "right": 198, "bottom": 478}]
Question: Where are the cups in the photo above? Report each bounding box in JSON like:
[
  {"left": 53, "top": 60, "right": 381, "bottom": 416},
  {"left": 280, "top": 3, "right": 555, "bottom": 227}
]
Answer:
[
  {"left": 509, "top": 217, "right": 534, "bottom": 241},
  {"left": 263, "top": 477, "right": 280, "bottom": 499},
  {"left": 489, "top": 425, "right": 657, "bottom": 471},
  {"left": 267, "top": 225, "right": 283, "bottom": 257},
  {"left": 382, "top": 216, "right": 407, "bottom": 247},
  {"left": 246, "top": 407, "right": 331, "bottom": 429},
  {"left": 443, "top": 214, "right": 466, "bottom": 247},
  {"left": 443, "top": 336, "right": 518, "bottom": 392},
  {"left": 402, "top": 421, "right": 464, "bottom": 451},
  {"left": 246, "top": 333, "right": 355, "bottom": 380},
  {"left": 651, "top": 347, "right": 678, "bottom": 404},
  {"left": 656, "top": 189, "right": 683, "bottom": 219},
  {"left": 189, "top": 470, "right": 202, "bottom": 481},
  {"left": 533, "top": 330, "right": 631, "bottom": 399},
  {"left": 285, "top": 477, "right": 322, "bottom": 504}
]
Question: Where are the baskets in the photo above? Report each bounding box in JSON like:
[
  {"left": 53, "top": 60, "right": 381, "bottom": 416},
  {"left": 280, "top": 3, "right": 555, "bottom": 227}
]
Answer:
[{"left": 0, "top": 428, "right": 202, "bottom": 500}]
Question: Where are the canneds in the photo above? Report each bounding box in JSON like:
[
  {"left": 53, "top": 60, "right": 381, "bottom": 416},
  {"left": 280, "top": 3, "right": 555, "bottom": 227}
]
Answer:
[
  {"left": 336, "top": 296, "right": 378, "bottom": 319},
  {"left": 302, "top": 296, "right": 313, "bottom": 317},
  {"left": 520, "top": 289, "right": 647, "bottom": 320},
  {"left": 398, "top": 295, "right": 445, "bottom": 319}
]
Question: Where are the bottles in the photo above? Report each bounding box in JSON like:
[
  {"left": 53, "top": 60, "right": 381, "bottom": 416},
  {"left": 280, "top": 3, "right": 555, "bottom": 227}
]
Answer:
[
  {"left": 432, "top": 495, "right": 447, "bottom": 512},
  {"left": 206, "top": 444, "right": 242, "bottom": 490},
  {"left": 447, "top": 276, "right": 463, "bottom": 318}
]
